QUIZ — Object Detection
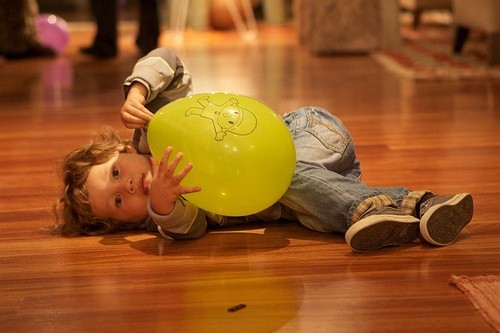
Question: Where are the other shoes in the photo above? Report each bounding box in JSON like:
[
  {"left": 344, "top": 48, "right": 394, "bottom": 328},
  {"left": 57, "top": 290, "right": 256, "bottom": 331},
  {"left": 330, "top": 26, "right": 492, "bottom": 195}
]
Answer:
[
  {"left": 81, "top": 46, "right": 115, "bottom": 59},
  {"left": 5, "top": 43, "right": 56, "bottom": 60}
]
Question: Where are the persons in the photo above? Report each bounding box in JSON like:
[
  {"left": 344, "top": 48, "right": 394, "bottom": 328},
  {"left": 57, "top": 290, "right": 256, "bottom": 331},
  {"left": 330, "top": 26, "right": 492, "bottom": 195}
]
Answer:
[
  {"left": 77, "top": 0, "right": 163, "bottom": 59},
  {"left": 41, "top": 47, "right": 474, "bottom": 252}
]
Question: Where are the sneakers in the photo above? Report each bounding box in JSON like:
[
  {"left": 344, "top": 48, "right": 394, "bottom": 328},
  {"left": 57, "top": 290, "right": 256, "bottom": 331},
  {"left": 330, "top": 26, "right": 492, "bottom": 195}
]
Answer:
[
  {"left": 419, "top": 192, "right": 474, "bottom": 247},
  {"left": 345, "top": 207, "right": 421, "bottom": 252}
]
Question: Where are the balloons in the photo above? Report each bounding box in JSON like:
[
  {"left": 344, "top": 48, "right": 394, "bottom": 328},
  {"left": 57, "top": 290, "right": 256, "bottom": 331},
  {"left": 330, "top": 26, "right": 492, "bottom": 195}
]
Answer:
[
  {"left": 36, "top": 14, "right": 71, "bottom": 47},
  {"left": 146, "top": 93, "right": 295, "bottom": 217}
]
